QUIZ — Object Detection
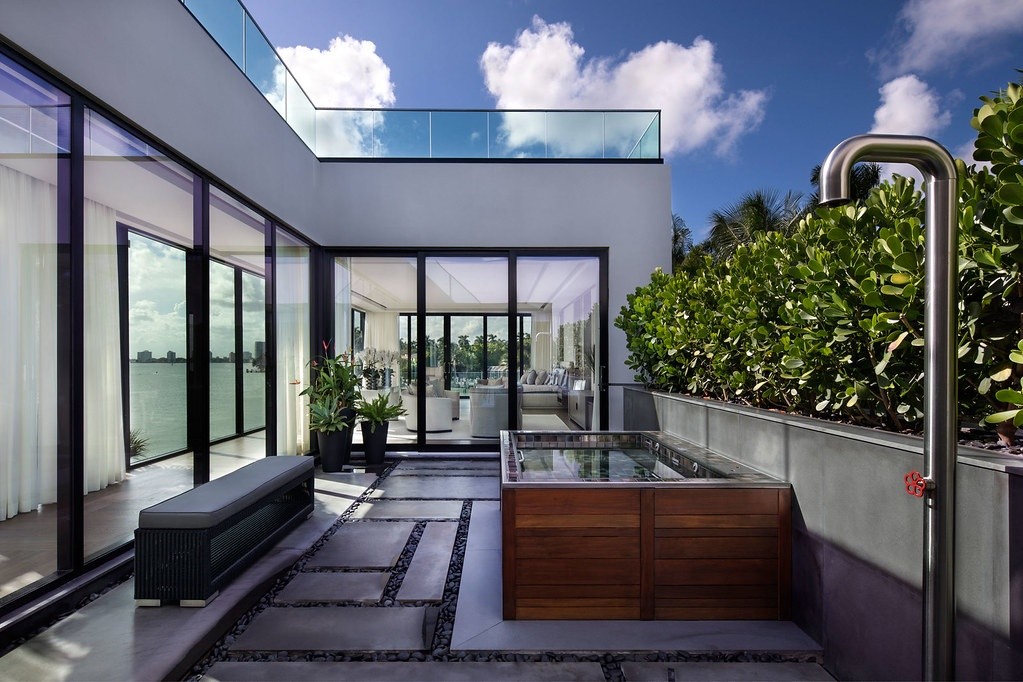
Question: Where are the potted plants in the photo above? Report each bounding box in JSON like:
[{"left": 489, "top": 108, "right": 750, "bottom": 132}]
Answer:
[
  {"left": 305, "top": 337, "right": 364, "bottom": 465},
  {"left": 306, "top": 395, "right": 349, "bottom": 473},
  {"left": 355, "top": 393, "right": 410, "bottom": 468}
]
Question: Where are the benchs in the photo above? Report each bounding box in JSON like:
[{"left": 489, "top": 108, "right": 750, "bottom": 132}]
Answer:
[{"left": 132, "top": 456, "right": 315, "bottom": 609}]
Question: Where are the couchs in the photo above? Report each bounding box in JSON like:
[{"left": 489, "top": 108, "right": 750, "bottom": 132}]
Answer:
[
  {"left": 468, "top": 386, "right": 523, "bottom": 438},
  {"left": 400, "top": 390, "right": 460, "bottom": 434}
]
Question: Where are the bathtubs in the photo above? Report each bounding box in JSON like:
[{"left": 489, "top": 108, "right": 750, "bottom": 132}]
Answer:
[{"left": 500, "top": 430, "right": 795, "bottom": 622}]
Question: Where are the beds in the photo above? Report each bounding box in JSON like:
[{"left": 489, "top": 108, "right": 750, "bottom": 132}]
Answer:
[{"left": 474, "top": 360, "right": 574, "bottom": 410}]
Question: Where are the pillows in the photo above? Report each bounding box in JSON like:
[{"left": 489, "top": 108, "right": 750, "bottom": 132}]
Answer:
[
  {"left": 477, "top": 379, "right": 488, "bottom": 385},
  {"left": 520, "top": 372, "right": 529, "bottom": 384},
  {"left": 561, "top": 370, "right": 569, "bottom": 386},
  {"left": 426, "top": 379, "right": 447, "bottom": 398},
  {"left": 553, "top": 368, "right": 566, "bottom": 386},
  {"left": 409, "top": 384, "right": 435, "bottom": 397},
  {"left": 406, "top": 385, "right": 414, "bottom": 395},
  {"left": 544, "top": 373, "right": 552, "bottom": 385},
  {"left": 535, "top": 369, "right": 547, "bottom": 385},
  {"left": 526, "top": 369, "right": 536, "bottom": 385},
  {"left": 487, "top": 376, "right": 502, "bottom": 386}
]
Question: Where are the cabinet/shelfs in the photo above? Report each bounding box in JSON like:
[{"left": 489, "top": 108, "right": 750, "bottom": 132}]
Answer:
[{"left": 567, "top": 389, "right": 594, "bottom": 431}]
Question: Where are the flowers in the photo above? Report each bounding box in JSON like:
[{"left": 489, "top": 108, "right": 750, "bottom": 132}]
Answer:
[{"left": 357, "top": 346, "right": 400, "bottom": 390}]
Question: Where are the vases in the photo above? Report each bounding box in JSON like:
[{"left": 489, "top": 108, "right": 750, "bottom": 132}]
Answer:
[{"left": 359, "top": 386, "right": 399, "bottom": 421}]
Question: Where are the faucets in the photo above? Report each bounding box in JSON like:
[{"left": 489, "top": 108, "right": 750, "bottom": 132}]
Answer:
[{"left": 817, "top": 131, "right": 961, "bottom": 682}]
